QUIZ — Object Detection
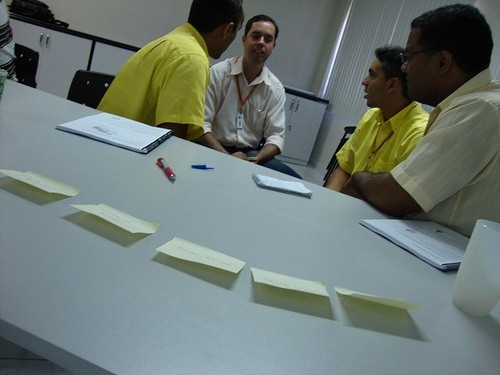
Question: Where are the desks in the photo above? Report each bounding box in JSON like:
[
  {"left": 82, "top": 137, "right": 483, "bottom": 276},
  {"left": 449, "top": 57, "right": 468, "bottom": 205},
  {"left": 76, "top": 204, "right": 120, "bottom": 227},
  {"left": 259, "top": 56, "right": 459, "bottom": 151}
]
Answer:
[{"left": 0, "top": 81, "right": 498, "bottom": 375}]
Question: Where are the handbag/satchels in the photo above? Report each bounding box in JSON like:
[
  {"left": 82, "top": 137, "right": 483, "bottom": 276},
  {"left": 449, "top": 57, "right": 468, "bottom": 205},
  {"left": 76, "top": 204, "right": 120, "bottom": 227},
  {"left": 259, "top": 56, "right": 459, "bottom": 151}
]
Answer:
[{"left": 10, "top": 0, "right": 55, "bottom": 24}]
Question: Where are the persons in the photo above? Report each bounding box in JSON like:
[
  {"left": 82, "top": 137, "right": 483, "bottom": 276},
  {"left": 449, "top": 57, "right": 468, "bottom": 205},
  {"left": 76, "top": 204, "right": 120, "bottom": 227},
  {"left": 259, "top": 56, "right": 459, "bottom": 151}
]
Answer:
[
  {"left": 98, "top": 0, "right": 245, "bottom": 140},
  {"left": 342, "top": 4, "right": 500, "bottom": 236},
  {"left": 0, "top": 0, "right": 18, "bottom": 82},
  {"left": 326, "top": 47, "right": 428, "bottom": 191},
  {"left": 203, "top": 15, "right": 302, "bottom": 180}
]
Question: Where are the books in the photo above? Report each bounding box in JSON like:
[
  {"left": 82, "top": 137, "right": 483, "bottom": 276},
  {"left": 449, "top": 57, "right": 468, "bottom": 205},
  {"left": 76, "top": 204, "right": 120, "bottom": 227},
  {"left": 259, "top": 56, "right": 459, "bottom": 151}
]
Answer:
[
  {"left": 360, "top": 219, "right": 470, "bottom": 270},
  {"left": 254, "top": 174, "right": 312, "bottom": 197},
  {"left": 57, "top": 112, "right": 175, "bottom": 153}
]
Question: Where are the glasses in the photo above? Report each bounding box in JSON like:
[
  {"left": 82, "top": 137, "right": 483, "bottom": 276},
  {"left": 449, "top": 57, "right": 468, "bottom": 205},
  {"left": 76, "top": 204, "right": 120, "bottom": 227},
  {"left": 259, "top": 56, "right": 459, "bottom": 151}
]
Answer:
[{"left": 399, "top": 45, "right": 444, "bottom": 65}]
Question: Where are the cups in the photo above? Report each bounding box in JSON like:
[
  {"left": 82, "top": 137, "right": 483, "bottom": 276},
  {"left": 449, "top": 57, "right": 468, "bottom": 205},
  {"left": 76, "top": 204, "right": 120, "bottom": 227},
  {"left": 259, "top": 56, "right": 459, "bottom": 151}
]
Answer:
[
  {"left": 0, "top": 69, "right": 8, "bottom": 99},
  {"left": 452, "top": 219, "right": 500, "bottom": 318}
]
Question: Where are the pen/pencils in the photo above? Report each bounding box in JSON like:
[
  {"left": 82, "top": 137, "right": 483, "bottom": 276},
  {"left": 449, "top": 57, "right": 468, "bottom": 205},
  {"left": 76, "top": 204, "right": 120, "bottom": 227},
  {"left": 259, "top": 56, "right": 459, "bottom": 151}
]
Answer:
[{"left": 157, "top": 157, "right": 176, "bottom": 180}]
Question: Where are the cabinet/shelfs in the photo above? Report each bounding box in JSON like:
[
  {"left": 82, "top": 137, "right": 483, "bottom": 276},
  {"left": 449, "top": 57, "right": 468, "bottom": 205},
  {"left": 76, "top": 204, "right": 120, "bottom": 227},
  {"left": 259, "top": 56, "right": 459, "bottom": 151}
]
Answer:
[
  {"left": 273, "top": 92, "right": 330, "bottom": 165},
  {"left": 5, "top": 17, "right": 92, "bottom": 99}
]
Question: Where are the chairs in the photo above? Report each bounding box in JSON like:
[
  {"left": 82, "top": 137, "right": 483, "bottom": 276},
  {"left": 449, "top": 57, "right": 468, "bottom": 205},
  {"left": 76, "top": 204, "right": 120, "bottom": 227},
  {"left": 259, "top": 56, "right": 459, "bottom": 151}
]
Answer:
[
  {"left": 68, "top": 68, "right": 117, "bottom": 110},
  {"left": 11, "top": 43, "right": 40, "bottom": 89}
]
